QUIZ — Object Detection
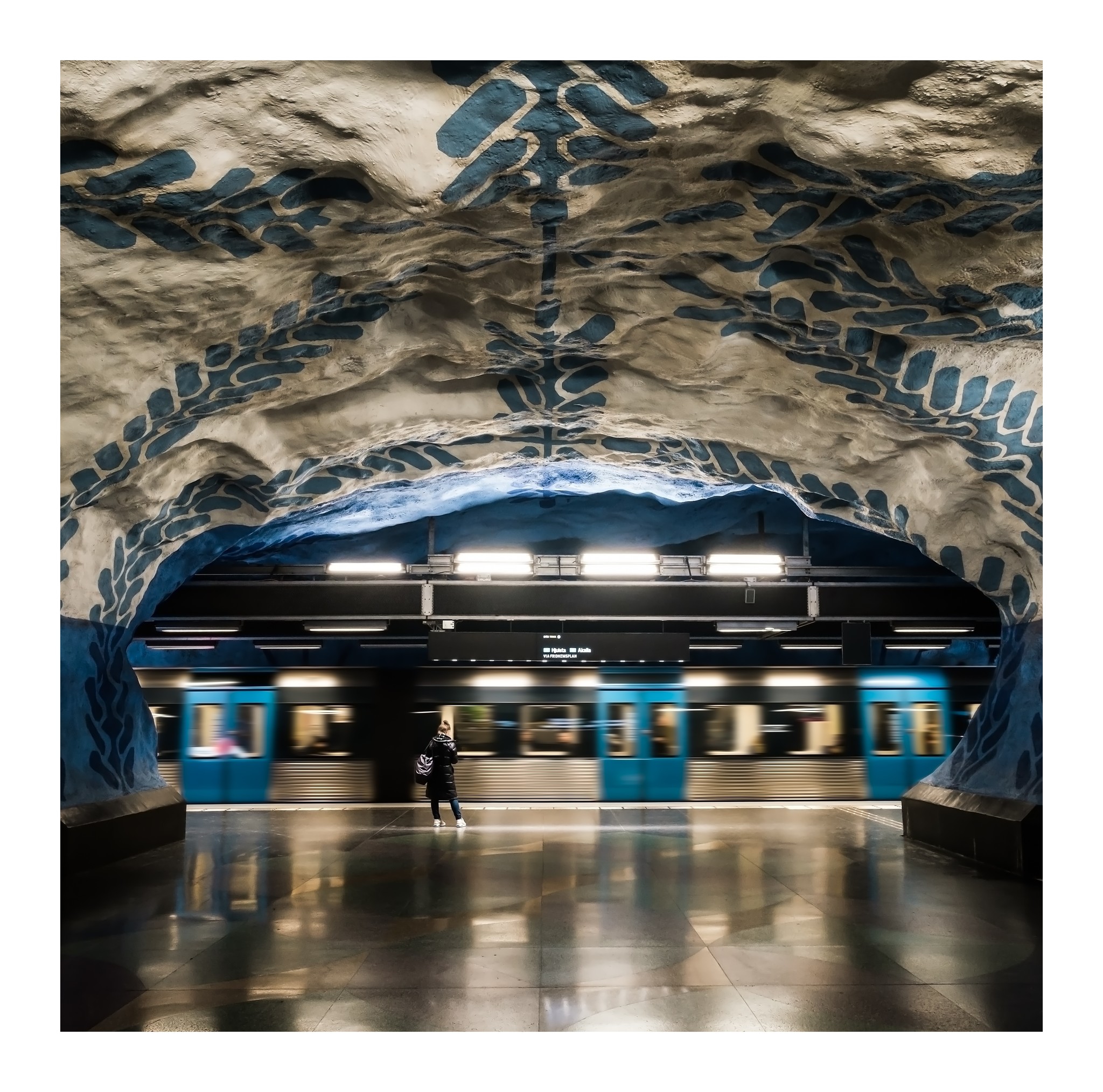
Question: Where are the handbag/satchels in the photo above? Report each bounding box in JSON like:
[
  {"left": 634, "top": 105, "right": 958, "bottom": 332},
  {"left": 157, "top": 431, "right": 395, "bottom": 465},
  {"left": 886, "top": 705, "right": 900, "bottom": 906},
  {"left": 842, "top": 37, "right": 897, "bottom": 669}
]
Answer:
[{"left": 415, "top": 753, "right": 434, "bottom": 785}]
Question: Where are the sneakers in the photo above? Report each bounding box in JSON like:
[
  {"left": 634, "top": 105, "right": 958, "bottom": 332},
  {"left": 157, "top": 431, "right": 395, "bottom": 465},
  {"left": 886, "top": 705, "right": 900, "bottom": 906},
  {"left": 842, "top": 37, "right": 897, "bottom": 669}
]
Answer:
[
  {"left": 434, "top": 819, "right": 446, "bottom": 827},
  {"left": 456, "top": 817, "right": 466, "bottom": 827}
]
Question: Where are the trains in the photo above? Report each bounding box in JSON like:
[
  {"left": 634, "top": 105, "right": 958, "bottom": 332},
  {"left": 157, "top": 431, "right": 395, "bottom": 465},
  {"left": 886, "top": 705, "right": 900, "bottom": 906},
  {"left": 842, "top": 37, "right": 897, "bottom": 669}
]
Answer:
[{"left": 133, "top": 663, "right": 995, "bottom": 804}]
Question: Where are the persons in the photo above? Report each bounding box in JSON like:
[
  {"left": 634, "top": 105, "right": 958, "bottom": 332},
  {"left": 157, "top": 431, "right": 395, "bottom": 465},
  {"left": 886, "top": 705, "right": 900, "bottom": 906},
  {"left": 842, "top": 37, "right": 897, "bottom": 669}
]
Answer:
[{"left": 425, "top": 719, "right": 468, "bottom": 828}]
